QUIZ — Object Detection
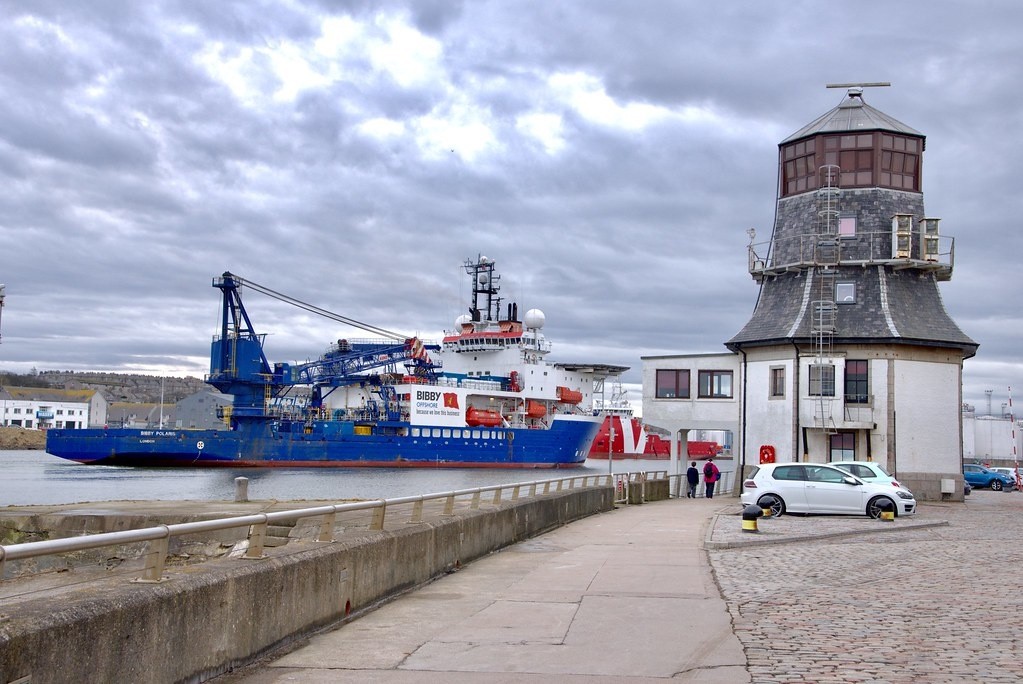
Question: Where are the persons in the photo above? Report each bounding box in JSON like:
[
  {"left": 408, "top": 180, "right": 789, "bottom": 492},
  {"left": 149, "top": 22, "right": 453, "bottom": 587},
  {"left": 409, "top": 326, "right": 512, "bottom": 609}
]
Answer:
[
  {"left": 687, "top": 462, "right": 699, "bottom": 498},
  {"left": 703, "top": 458, "right": 719, "bottom": 500}
]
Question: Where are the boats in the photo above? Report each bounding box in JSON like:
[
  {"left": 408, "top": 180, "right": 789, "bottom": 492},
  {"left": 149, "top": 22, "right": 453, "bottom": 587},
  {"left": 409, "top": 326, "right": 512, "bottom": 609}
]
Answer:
[
  {"left": 557, "top": 386, "right": 583, "bottom": 404},
  {"left": 45, "top": 253, "right": 631, "bottom": 470},
  {"left": 466, "top": 408, "right": 502, "bottom": 427},
  {"left": 588, "top": 374, "right": 718, "bottom": 460},
  {"left": 519, "top": 400, "right": 546, "bottom": 419}
]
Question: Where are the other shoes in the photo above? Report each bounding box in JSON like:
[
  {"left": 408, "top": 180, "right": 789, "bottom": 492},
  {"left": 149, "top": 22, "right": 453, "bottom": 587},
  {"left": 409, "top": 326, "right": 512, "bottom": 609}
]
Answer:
[
  {"left": 691, "top": 496, "right": 695, "bottom": 498},
  {"left": 686, "top": 493, "right": 690, "bottom": 499},
  {"left": 709, "top": 497, "right": 712, "bottom": 501}
]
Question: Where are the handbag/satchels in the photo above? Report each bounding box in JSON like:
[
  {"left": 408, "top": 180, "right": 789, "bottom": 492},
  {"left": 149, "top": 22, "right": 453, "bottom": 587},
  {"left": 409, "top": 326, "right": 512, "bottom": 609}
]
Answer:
[{"left": 715, "top": 473, "right": 721, "bottom": 481}]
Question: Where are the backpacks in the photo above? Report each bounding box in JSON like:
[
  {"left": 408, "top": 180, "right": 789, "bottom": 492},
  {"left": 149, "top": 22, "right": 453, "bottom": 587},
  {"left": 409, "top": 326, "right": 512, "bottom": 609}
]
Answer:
[{"left": 705, "top": 466, "right": 713, "bottom": 478}]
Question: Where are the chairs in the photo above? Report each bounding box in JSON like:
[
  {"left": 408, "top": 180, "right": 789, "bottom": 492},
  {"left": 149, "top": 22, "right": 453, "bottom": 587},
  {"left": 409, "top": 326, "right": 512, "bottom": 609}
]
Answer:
[{"left": 810, "top": 471, "right": 818, "bottom": 481}]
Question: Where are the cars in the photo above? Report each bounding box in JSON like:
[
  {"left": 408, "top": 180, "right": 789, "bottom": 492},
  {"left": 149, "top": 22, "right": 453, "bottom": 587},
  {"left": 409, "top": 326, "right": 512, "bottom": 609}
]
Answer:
[
  {"left": 988, "top": 467, "right": 1023, "bottom": 487},
  {"left": 740, "top": 463, "right": 917, "bottom": 519},
  {"left": 807, "top": 461, "right": 901, "bottom": 488},
  {"left": 963, "top": 464, "right": 1016, "bottom": 491}
]
